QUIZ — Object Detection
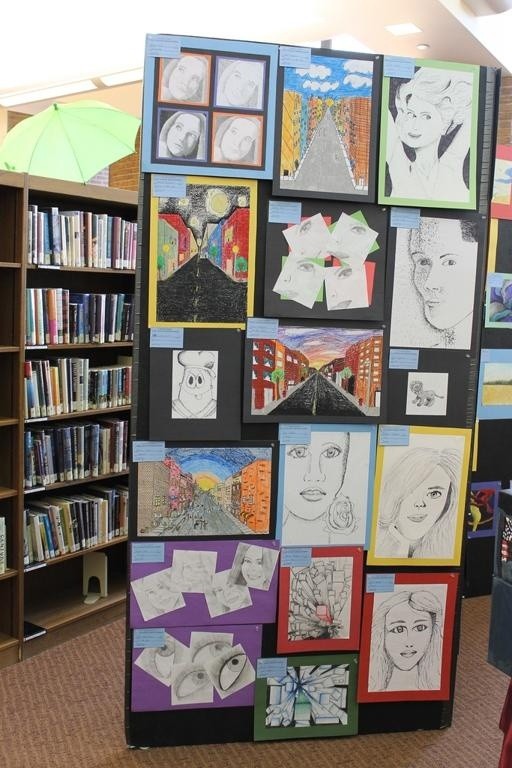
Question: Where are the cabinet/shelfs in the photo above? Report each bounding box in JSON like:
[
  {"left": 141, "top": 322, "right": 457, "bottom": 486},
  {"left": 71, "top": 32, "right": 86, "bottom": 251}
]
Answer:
[
  {"left": 15, "top": 171, "right": 140, "bottom": 661},
  {"left": 0, "top": 171, "right": 24, "bottom": 668}
]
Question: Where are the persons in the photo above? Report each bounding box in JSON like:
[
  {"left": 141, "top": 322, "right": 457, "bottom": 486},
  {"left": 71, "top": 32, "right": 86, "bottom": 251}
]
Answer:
[
  {"left": 171, "top": 549, "right": 214, "bottom": 590},
  {"left": 375, "top": 444, "right": 460, "bottom": 563},
  {"left": 160, "top": 52, "right": 208, "bottom": 103},
  {"left": 322, "top": 263, "right": 370, "bottom": 312},
  {"left": 141, "top": 569, "right": 178, "bottom": 615},
  {"left": 327, "top": 219, "right": 377, "bottom": 262},
  {"left": 211, "top": 115, "right": 261, "bottom": 166},
  {"left": 215, "top": 58, "right": 264, "bottom": 111},
  {"left": 204, "top": 570, "right": 253, "bottom": 615},
  {"left": 366, "top": 587, "right": 444, "bottom": 691},
  {"left": 229, "top": 541, "right": 277, "bottom": 589},
  {"left": 157, "top": 110, "right": 203, "bottom": 162},
  {"left": 274, "top": 261, "right": 318, "bottom": 308},
  {"left": 384, "top": 69, "right": 474, "bottom": 201},
  {"left": 285, "top": 218, "right": 327, "bottom": 259},
  {"left": 403, "top": 216, "right": 481, "bottom": 351},
  {"left": 284, "top": 430, "right": 357, "bottom": 542}
]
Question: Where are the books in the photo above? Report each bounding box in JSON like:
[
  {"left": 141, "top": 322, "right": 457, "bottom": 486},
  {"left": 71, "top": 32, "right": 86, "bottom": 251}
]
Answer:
[
  {"left": 26, "top": 288, "right": 135, "bottom": 346},
  {"left": 24, "top": 355, "right": 133, "bottom": 420},
  {"left": 22, "top": 619, "right": 47, "bottom": 644},
  {"left": 23, "top": 484, "right": 130, "bottom": 564},
  {"left": 24, "top": 416, "right": 128, "bottom": 487},
  {"left": 0, "top": 514, "right": 8, "bottom": 576},
  {"left": 28, "top": 205, "right": 137, "bottom": 270}
]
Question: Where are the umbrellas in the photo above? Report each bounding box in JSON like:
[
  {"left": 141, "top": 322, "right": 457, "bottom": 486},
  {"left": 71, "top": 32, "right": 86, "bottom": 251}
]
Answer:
[{"left": 0, "top": 100, "right": 142, "bottom": 185}]
